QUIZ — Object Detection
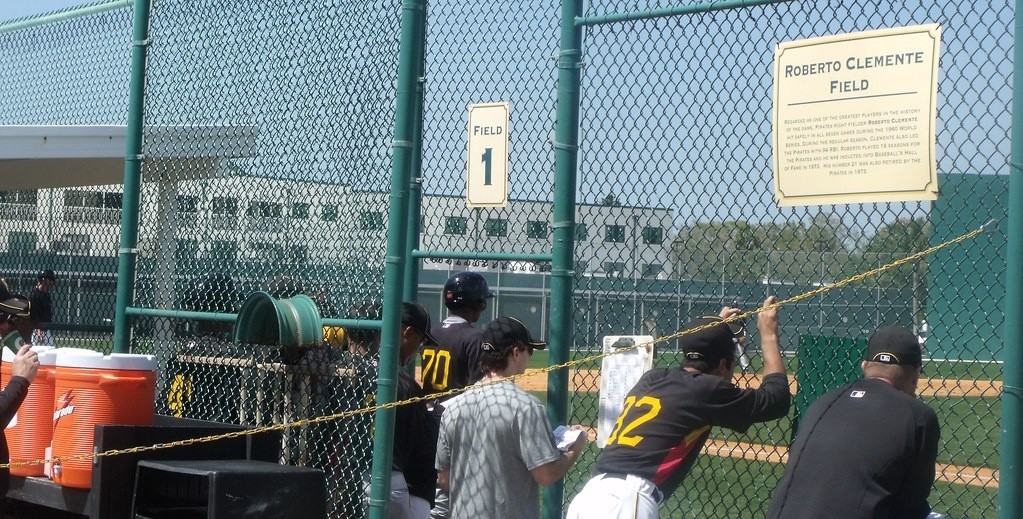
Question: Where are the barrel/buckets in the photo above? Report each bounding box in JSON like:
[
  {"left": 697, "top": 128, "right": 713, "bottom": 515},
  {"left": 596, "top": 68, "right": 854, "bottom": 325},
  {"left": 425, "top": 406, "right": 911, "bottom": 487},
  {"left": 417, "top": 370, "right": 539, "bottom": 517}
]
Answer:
[
  {"left": 2, "top": 346, "right": 55, "bottom": 476},
  {"left": 55, "top": 348, "right": 156, "bottom": 489}
]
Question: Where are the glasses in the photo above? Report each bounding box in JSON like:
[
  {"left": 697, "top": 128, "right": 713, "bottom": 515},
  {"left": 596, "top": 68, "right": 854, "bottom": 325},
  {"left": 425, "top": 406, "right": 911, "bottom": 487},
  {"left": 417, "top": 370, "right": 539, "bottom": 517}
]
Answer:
[
  {"left": 0, "top": 312, "right": 12, "bottom": 321},
  {"left": 412, "top": 328, "right": 427, "bottom": 346},
  {"left": 518, "top": 347, "right": 533, "bottom": 356}
]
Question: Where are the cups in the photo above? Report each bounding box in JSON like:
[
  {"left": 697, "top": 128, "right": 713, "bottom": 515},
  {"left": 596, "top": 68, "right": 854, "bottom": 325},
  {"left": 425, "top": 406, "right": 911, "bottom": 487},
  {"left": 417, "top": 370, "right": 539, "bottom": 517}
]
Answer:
[{"left": 1, "top": 330, "right": 24, "bottom": 356}]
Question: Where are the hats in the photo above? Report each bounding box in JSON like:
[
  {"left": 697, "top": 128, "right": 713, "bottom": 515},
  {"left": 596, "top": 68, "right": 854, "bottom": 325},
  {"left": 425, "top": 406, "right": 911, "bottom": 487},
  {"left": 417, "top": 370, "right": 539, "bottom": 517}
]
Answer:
[
  {"left": 480, "top": 316, "right": 547, "bottom": 351},
  {"left": 0, "top": 277, "right": 31, "bottom": 317},
  {"left": 401, "top": 302, "right": 439, "bottom": 347},
  {"left": 866, "top": 323, "right": 923, "bottom": 374},
  {"left": 37, "top": 269, "right": 56, "bottom": 279},
  {"left": 682, "top": 315, "right": 743, "bottom": 361}
]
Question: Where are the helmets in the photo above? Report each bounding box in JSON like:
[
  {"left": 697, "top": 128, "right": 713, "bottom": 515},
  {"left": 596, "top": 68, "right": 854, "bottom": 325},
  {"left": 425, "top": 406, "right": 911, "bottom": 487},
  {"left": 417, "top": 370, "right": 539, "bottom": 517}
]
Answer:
[{"left": 443, "top": 272, "right": 495, "bottom": 303}]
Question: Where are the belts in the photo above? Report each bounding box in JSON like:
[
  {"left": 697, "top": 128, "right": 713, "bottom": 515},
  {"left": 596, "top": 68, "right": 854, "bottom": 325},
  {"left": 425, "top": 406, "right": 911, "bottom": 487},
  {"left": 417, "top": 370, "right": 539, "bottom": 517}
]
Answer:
[{"left": 601, "top": 472, "right": 661, "bottom": 503}]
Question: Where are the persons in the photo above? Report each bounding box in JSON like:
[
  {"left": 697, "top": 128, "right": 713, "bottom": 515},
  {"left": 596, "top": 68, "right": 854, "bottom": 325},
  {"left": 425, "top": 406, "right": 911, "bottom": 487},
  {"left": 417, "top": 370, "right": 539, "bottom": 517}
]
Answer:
[
  {"left": 153, "top": 259, "right": 343, "bottom": 468},
  {"left": 344, "top": 298, "right": 436, "bottom": 506},
  {"left": 763, "top": 323, "right": 941, "bottom": 519},
  {"left": 435, "top": 315, "right": 586, "bottom": 519},
  {"left": 565, "top": 295, "right": 791, "bottom": 519},
  {"left": 29, "top": 268, "right": 56, "bottom": 347},
  {"left": 419, "top": 267, "right": 499, "bottom": 412},
  {"left": 0, "top": 276, "right": 42, "bottom": 501}
]
